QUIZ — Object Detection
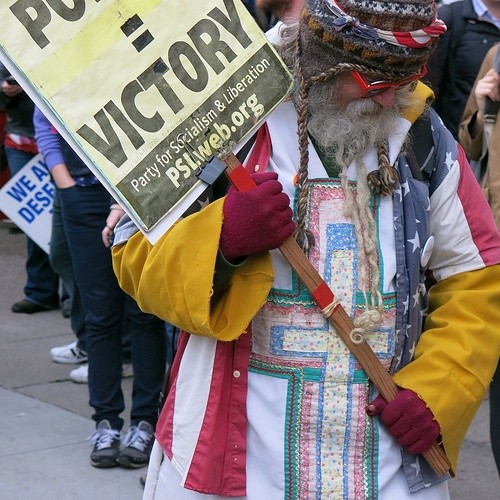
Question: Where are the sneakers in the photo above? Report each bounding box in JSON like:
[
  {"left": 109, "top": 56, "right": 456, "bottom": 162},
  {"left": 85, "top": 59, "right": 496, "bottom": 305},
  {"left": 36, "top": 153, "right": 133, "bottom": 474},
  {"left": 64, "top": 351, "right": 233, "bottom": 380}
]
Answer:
[
  {"left": 86, "top": 420, "right": 120, "bottom": 465},
  {"left": 71, "top": 363, "right": 135, "bottom": 381},
  {"left": 50, "top": 341, "right": 89, "bottom": 362},
  {"left": 117, "top": 420, "right": 155, "bottom": 467}
]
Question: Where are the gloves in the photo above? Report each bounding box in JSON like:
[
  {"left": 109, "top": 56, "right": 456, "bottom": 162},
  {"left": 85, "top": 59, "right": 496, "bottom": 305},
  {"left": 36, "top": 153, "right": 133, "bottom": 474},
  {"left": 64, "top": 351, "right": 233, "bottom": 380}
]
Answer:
[
  {"left": 219, "top": 172, "right": 296, "bottom": 259},
  {"left": 367, "top": 386, "right": 440, "bottom": 454}
]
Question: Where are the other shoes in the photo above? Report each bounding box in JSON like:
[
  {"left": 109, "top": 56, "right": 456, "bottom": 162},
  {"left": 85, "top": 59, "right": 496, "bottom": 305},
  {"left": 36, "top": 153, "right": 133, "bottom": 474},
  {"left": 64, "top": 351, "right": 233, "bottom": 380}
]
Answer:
[
  {"left": 12, "top": 299, "right": 61, "bottom": 313},
  {"left": 61, "top": 296, "right": 71, "bottom": 316}
]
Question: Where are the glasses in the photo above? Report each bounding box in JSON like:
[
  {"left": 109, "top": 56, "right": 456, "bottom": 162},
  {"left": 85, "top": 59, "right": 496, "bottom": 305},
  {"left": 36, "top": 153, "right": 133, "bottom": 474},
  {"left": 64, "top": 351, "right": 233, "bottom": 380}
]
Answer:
[{"left": 350, "top": 67, "right": 427, "bottom": 99}]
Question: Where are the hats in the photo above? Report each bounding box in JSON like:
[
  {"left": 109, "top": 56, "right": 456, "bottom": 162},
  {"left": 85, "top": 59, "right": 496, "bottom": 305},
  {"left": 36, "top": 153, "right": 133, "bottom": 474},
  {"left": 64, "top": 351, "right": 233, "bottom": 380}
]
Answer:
[{"left": 295, "top": 0, "right": 445, "bottom": 257}]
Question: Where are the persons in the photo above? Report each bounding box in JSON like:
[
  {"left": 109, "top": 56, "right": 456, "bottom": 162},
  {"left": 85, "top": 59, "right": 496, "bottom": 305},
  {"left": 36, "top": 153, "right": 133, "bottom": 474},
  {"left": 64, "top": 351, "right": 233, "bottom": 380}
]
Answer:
[
  {"left": 0, "top": 0, "right": 500, "bottom": 500},
  {"left": 34, "top": 104, "right": 167, "bottom": 468}
]
